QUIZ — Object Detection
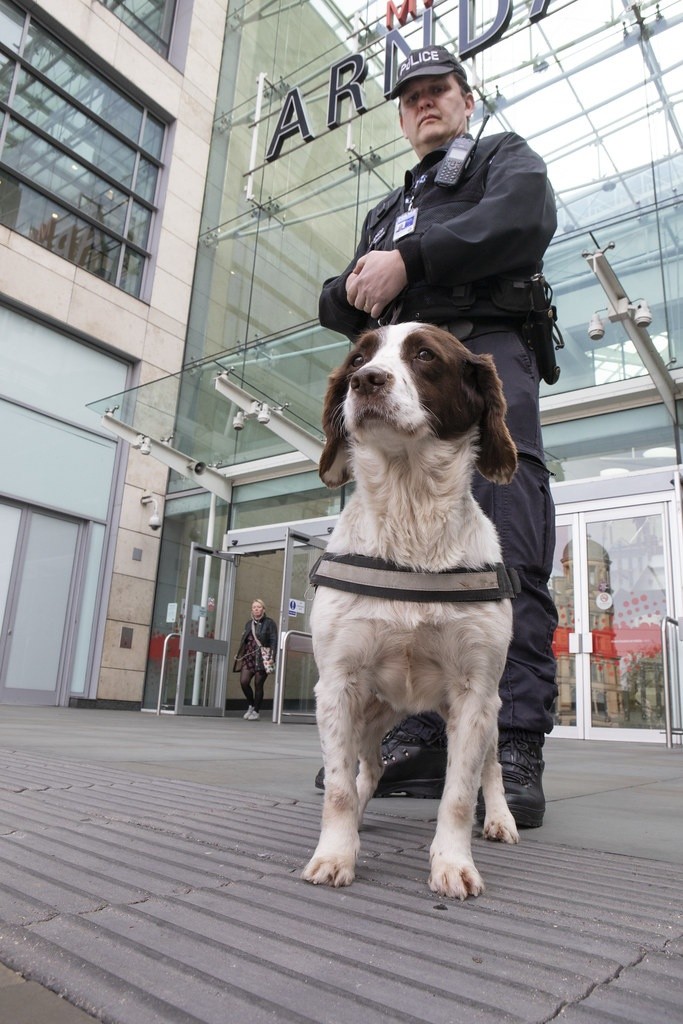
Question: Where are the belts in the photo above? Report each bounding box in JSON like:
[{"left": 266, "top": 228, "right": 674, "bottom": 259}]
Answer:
[{"left": 439, "top": 319, "right": 512, "bottom": 341}]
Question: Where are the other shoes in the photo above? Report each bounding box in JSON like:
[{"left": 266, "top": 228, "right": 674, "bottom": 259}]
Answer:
[
  {"left": 248, "top": 711, "right": 260, "bottom": 720},
  {"left": 244, "top": 705, "right": 255, "bottom": 719}
]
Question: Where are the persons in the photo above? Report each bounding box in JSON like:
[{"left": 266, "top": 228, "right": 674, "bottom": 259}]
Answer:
[
  {"left": 315, "top": 45, "right": 559, "bottom": 828},
  {"left": 232, "top": 599, "right": 277, "bottom": 720}
]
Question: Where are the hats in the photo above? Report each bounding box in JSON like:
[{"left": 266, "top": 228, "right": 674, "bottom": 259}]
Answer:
[{"left": 390, "top": 45, "right": 467, "bottom": 99}]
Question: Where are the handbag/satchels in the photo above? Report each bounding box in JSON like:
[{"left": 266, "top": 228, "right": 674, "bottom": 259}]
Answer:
[{"left": 260, "top": 647, "right": 275, "bottom": 673}]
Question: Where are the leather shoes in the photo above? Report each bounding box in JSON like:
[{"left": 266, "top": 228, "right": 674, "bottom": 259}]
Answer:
[
  {"left": 315, "top": 729, "right": 447, "bottom": 799},
  {"left": 476, "top": 740, "right": 545, "bottom": 827}
]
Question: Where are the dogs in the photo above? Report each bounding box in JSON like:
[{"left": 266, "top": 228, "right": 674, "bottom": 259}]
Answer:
[{"left": 299, "top": 321, "right": 522, "bottom": 902}]
